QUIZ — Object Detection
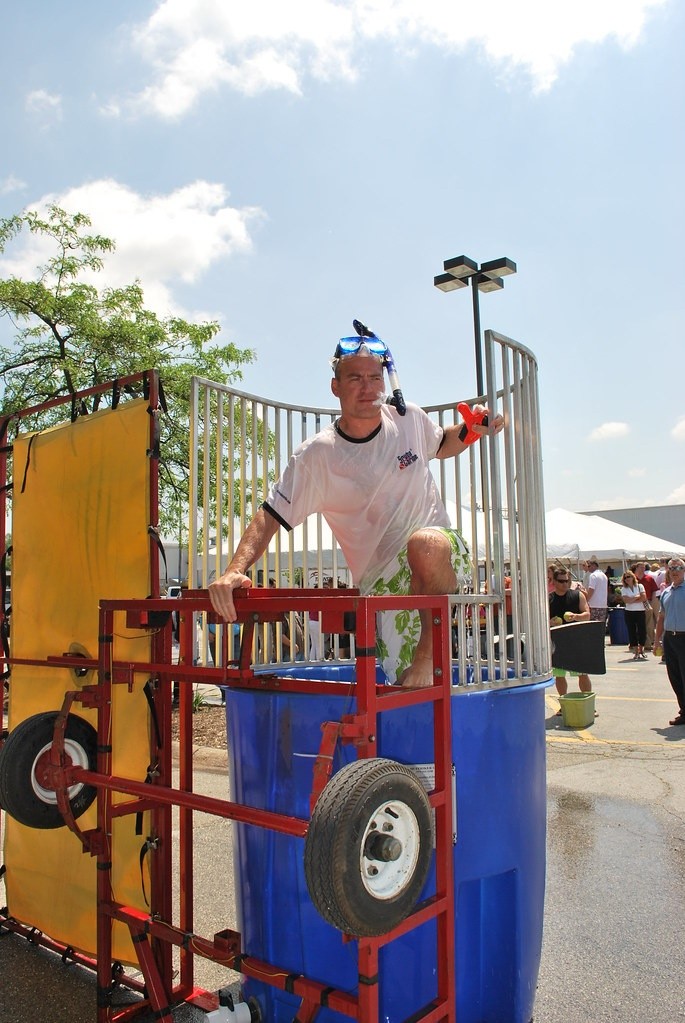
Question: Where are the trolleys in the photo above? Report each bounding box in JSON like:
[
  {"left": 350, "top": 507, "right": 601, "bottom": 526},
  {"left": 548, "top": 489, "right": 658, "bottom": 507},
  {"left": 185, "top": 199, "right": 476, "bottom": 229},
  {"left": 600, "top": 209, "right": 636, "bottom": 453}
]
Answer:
[{"left": 1, "top": 585, "right": 460, "bottom": 1023}]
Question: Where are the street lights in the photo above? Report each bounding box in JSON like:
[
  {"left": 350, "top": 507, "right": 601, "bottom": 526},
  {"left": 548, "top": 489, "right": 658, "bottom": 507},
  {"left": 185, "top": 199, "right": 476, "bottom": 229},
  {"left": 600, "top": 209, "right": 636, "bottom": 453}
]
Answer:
[{"left": 429, "top": 253, "right": 517, "bottom": 511}]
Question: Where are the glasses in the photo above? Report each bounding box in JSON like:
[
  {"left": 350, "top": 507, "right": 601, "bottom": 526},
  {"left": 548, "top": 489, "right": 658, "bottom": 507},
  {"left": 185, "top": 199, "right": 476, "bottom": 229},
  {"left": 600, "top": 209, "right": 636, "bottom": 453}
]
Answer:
[
  {"left": 624, "top": 576, "right": 632, "bottom": 580},
  {"left": 334, "top": 337, "right": 388, "bottom": 357},
  {"left": 556, "top": 579, "right": 569, "bottom": 583},
  {"left": 668, "top": 566, "right": 685, "bottom": 571}
]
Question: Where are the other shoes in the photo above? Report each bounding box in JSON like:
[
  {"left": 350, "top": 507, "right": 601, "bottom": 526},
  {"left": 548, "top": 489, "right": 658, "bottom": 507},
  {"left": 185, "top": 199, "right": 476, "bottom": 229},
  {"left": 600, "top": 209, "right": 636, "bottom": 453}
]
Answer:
[
  {"left": 639, "top": 651, "right": 647, "bottom": 658},
  {"left": 595, "top": 710, "right": 599, "bottom": 717},
  {"left": 659, "top": 661, "right": 667, "bottom": 664},
  {"left": 669, "top": 715, "right": 685, "bottom": 725},
  {"left": 633, "top": 653, "right": 640, "bottom": 659},
  {"left": 556, "top": 708, "right": 563, "bottom": 715}
]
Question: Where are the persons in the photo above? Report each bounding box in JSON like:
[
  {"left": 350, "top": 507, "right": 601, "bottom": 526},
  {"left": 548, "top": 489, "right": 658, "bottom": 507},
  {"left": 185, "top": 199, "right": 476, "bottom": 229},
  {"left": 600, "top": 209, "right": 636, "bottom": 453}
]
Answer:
[
  {"left": 207, "top": 343, "right": 505, "bottom": 689},
  {"left": 160, "top": 577, "right": 351, "bottom": 712},
  {"left": 452, "top": 557, "right": 685, "bottom": 725}
]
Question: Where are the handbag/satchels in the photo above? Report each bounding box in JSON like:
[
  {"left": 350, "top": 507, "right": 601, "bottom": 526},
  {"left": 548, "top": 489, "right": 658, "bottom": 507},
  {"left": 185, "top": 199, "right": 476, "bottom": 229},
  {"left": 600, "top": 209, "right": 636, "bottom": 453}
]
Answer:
[{"left": 639, "top": 585, "right": 653, "bottom": 611}]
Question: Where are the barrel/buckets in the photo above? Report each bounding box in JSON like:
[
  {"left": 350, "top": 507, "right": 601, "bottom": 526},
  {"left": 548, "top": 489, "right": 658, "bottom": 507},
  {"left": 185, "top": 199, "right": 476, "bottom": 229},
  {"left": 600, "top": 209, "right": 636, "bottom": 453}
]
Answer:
[
  {"left": 221, "top": 665, "right": 556, "bottom": 1022},
  {"left": 608, "top": 607, "right": 629, "bottom": 644}
]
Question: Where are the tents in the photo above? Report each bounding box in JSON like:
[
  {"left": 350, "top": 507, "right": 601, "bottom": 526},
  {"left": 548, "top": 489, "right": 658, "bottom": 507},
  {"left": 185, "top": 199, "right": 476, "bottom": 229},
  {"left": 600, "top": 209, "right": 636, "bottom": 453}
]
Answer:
[{"left": 196, "top": 497, "right": 685, "bottom": 571}]
